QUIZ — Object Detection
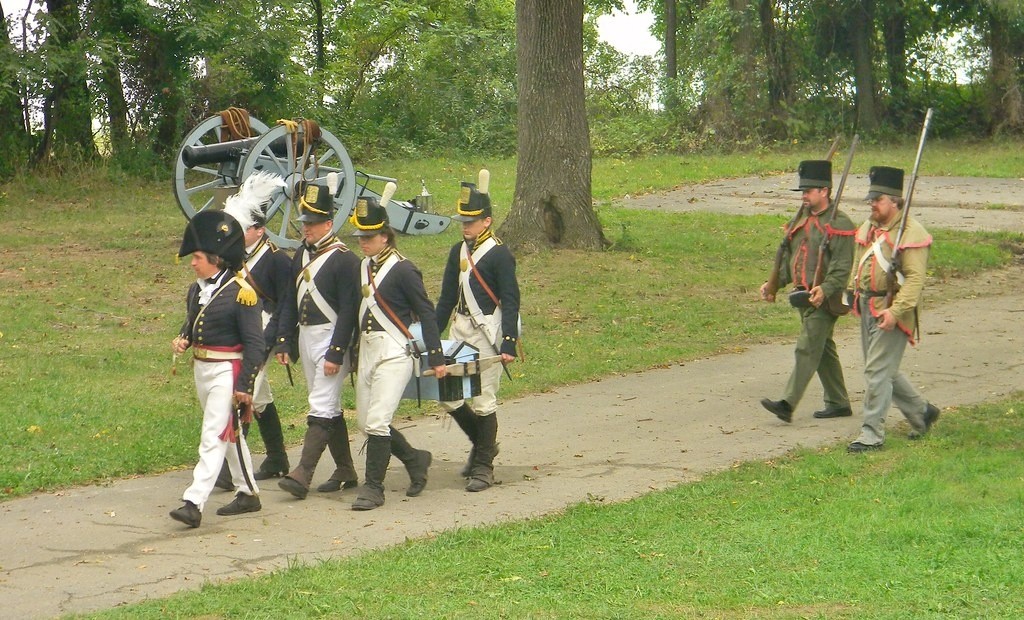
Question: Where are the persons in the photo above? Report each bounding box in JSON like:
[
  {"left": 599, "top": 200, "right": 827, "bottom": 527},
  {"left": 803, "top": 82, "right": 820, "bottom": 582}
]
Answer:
[
  {"left": 435, "top": 182, "right": 520, "bottom": 492},
  {"left": 846, "top": 166, "right": 941, "bottom": 453},
  {"left": 351, "top": 196, "right": 446, "bottom": 511},
  {"left": 275, "top": 184, "right": 362, "bottom": 500},
  {"left": 215, "top": 200, "right": 300, "bottom": 491},
  {"left": 169, "top": 210, "right": 266, "bottom": 529},
  {"left": 760, "top": 160, "right": 856, "bottom": 422}
]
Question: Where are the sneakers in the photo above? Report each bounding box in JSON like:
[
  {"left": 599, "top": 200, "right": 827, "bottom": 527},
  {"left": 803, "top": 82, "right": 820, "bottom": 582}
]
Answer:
[
  {"left": 216, "top": 492, "right": 262, "bottom": 516},
  {"left": 759, "top": 397, "right": 793, "bottom": 423},
  {"left": 812, "top": 404, "right": 852, "bottom": 419},
  {"left": 846, "top": 440, "right": 886, "bottom": 452},
  {"left": 909, "top": 402, "right": 941, "bottom": 440},
  {"left": 170, "top": 500, "right": 202, "bottom": 529}
]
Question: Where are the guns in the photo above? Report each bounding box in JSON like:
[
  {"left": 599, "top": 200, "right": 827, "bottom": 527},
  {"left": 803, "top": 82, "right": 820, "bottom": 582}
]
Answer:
[
  {"left": 810, "top": 132, "right": 859, "bottom": 301},
  {"left": 761, "top": 136, "right": 841, "bottom": 303},
  {"left": 881, "top": 106, "right": 935, "bottom": 325}
]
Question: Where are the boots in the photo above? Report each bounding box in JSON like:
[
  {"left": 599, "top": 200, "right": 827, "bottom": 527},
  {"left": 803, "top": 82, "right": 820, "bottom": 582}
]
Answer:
[
  {"left": 317, "top": 409, "right": 358, "bottom": 493},
  {"left": 447, "top": 400, "right": 500, "bottom": 477},
  {"left": 277, "top": 414, "right": 340, "bottom": 500},
  {"left": 465, "top": 409, "right": 497, "bottom": 492},
  {"left": 350, "top": 434, "right": 391, "bottom": 510},
  {"left": 245, "top": 401, "right": 290, "bottom": 480},
  {"left": 212, "top": 419, "right": 250, "bottom": 492},
  {"left": 387, "top": 425, "right": 432, "bottom": 496}
]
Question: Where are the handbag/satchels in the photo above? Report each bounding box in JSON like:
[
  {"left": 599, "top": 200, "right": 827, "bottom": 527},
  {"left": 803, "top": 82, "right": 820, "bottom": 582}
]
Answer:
[
  {"left": 823, "top": 288, "right": 850, "bottom": 316},
  {"left": 785, "top": 286, "right": 812, "bottom": 308}
]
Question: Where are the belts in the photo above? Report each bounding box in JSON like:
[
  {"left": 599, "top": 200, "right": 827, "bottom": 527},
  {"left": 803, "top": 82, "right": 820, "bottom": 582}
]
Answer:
[
  {"left": 192, "top": 347, "right": 243, "bottom": 359},
  {"left": 858, "top": 287, "right": 900, "bottom": 297}
]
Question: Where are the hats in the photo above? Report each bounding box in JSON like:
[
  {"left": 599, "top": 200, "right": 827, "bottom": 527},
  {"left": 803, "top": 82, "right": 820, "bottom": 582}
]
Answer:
[
  {"left": 349, "top": 181, "right": 397, "bottom": 236},
  {"left": 862, "top": 166, "right": 904, "bottom": 201},
  {"left": 249, "top": 202, "right": 269, "bottom": 228},
  {"left": 788, "top": 160, "right": 832, "bottom": 190},
  {"left": 451, "top": 169, "right": 492, "bottom": 222},
  {"left": 178, "top": 171, "right": 290, "bottom": 267},
  {"left": 294, "top": 171, "right": 338, "bottom": 222}
]
